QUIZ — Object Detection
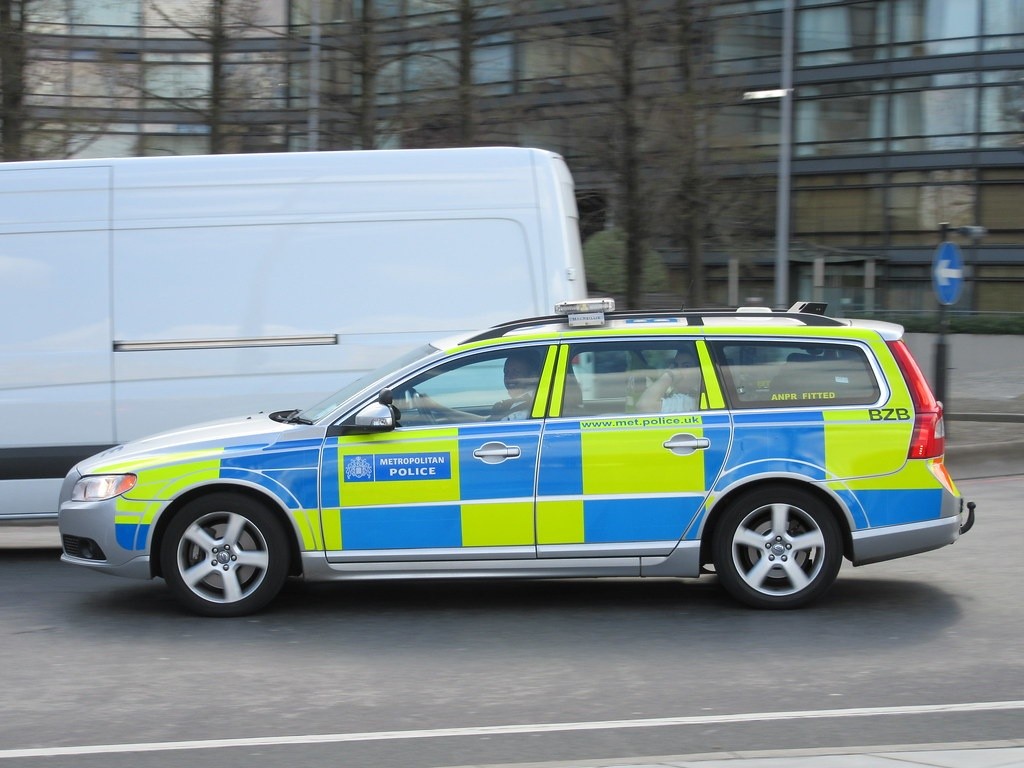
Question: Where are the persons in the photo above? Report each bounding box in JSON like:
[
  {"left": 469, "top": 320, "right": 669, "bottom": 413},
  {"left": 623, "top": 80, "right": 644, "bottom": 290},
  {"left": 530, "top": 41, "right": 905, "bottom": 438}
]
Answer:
[
  {"left": 420, "top": 350, "right": 540, "bottom": 422},
  {"left": 636, "top": 350, "right": 699, "bottom": 416}
]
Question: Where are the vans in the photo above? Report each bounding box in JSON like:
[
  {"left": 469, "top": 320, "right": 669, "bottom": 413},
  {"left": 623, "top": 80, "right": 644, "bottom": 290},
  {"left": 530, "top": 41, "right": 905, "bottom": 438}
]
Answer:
[{"left": 0, "top": 145, "right": 595, "bottom": 521}]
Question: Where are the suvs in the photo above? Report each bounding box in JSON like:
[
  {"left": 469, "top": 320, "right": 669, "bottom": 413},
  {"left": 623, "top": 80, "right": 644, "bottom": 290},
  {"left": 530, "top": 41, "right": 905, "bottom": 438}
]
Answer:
[{"left": 56, "top": 298, "right": 976, "bottom": 618}]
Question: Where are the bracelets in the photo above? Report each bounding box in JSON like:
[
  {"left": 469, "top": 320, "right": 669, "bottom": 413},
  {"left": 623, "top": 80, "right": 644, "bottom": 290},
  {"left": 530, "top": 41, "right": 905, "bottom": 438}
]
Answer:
[{"left": 661, "top": 371, "right": 674, "bottom": 381}]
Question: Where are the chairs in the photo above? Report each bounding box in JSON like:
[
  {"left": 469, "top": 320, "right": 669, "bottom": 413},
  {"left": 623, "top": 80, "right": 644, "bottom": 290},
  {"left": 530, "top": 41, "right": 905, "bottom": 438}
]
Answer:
[{"left": 564, "top": 369, "right": 583, "bottom": 417}]
